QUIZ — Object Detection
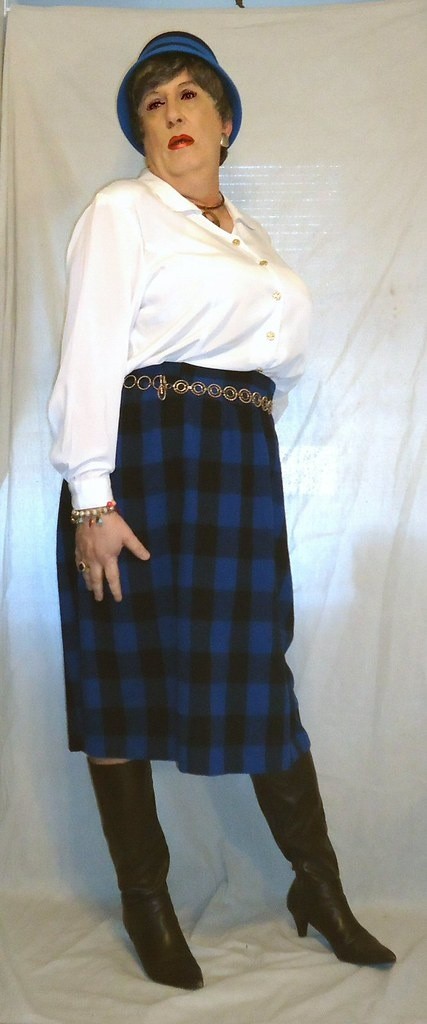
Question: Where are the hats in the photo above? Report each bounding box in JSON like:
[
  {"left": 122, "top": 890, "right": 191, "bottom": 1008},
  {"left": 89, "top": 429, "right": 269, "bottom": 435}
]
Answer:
[{"left": 116, "top": 31, "right": 242, "bottom": 157}]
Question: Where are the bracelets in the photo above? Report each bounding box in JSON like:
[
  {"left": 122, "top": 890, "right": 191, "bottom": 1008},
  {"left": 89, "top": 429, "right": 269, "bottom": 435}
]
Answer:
[{"left": 70, "top": 501, "right": 117, "bottom": 527}]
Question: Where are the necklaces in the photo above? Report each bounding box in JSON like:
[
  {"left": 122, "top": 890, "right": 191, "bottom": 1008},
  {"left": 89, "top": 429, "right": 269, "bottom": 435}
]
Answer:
[{"left": 182, "top": 190, "right": 225, "bottom": 227}]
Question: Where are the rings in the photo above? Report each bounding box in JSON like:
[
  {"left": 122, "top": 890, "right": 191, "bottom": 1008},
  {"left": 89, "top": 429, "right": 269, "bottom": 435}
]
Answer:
[{"left": 78, "top": 560, "right": 90, "bottom": 574}]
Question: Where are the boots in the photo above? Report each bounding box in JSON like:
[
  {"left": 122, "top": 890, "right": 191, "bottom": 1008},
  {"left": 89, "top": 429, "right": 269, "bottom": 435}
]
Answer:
[
  {"left": 86, "top": 757, "right": 203, "bottom": 991},
  {"left": 251, "top": 748, "right": 397, "bottom": 968}
]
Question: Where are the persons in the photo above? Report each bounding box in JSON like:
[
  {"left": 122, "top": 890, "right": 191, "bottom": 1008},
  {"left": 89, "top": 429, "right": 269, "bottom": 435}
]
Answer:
[{"left": 47, "top": 29, "right": 398, "bottom": 991}]
